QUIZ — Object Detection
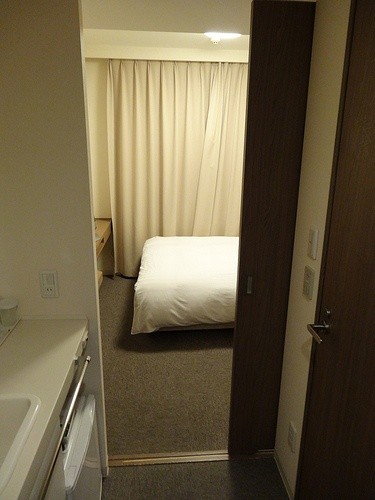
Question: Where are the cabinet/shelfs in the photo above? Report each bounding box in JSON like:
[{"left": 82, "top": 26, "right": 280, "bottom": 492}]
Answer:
[{"left": 39, "top": 344, "right": 104, "bottom": 500}]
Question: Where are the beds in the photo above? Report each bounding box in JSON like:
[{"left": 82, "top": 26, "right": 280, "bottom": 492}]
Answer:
[{"left": 130, "top": 235, "right": 239, "bottom": 336}]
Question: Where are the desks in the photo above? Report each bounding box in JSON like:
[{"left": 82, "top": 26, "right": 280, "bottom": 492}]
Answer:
[{"left": 95, "top": 218, "right": 112, "bottom": 261}]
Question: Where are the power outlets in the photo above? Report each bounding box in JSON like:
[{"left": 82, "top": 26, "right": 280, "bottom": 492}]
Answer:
[{"left": 40, "top": 269, "right": 59, "bottom": 298}]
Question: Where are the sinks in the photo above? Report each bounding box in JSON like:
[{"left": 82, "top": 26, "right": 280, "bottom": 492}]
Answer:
[{"left": 0, "top": 392, "right": 40, "bottom": 494}]
